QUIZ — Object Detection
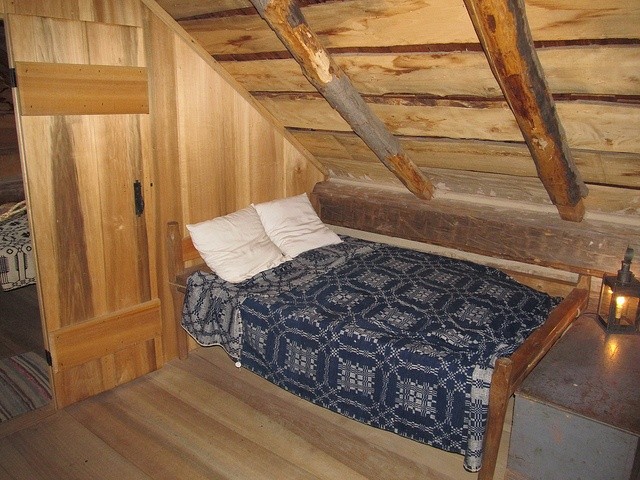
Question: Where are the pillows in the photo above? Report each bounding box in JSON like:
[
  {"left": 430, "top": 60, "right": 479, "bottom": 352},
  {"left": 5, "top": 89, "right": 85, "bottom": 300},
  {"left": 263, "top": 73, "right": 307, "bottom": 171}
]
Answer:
[
  {"left": 184, "top": 206, "right": 291, "bottom": 283},
  {"left": 253, "top": 192, "right": 346, "bottom": 262}
]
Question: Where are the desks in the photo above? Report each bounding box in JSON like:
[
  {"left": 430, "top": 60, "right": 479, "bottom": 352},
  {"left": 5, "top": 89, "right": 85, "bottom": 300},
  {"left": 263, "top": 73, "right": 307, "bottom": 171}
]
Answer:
[{"left": 505, "top": 313, "right": 639, "bottom": 480}]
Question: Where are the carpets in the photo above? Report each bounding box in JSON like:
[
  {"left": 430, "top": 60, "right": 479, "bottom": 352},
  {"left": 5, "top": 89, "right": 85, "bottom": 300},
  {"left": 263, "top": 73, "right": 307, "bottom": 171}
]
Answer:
[{"left": 0, "top": 349, "right": 55, "bottom": 427}]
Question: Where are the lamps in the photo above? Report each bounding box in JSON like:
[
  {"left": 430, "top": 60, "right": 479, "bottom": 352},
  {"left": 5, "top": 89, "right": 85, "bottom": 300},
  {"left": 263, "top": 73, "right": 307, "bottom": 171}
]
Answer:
[{"left": 596, "top": 245, "right": 640, "bottom": 339}]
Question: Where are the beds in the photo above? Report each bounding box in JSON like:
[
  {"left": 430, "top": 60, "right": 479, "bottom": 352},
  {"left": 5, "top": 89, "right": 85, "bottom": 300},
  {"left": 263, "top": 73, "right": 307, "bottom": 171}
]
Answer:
[{"left": 166, "top": 221, "right": 592, "bottom": 479}]
